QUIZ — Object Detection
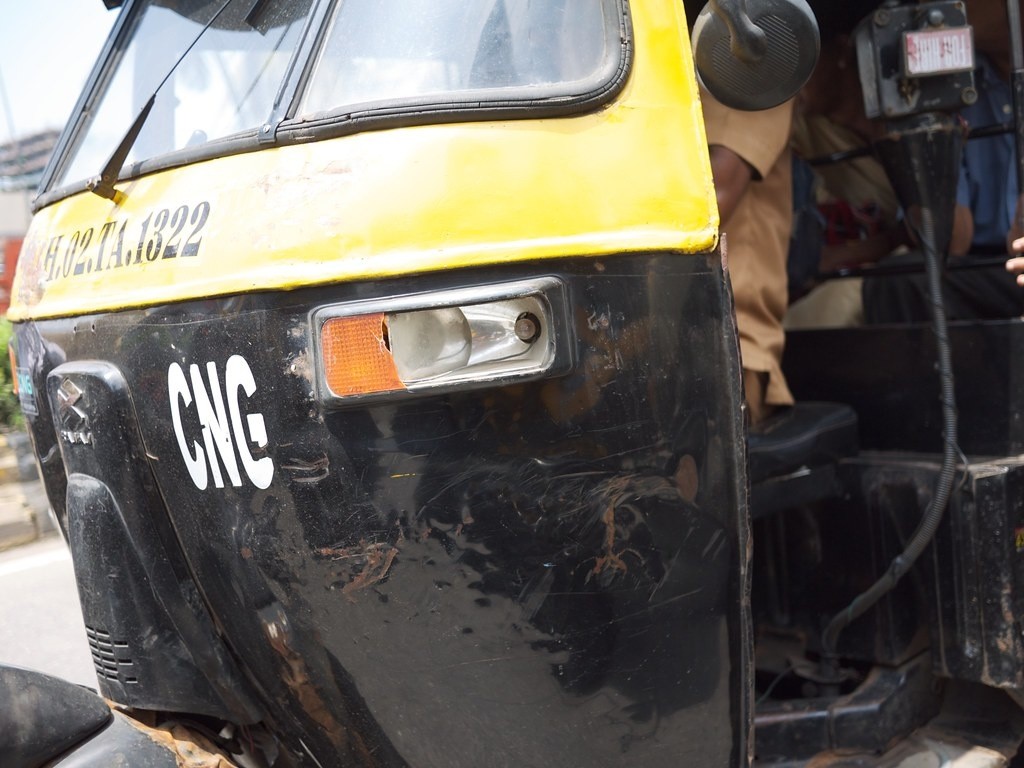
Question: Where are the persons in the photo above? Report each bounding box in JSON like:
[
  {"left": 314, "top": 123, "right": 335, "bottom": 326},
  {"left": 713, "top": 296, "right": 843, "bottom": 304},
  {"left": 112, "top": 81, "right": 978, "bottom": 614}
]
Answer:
[
  {"left": 509, "top": 0, "right": 795, "bottom": 439},
  {"left": 907, "top": 0, "right": 1024, "bottom": 296}
]
